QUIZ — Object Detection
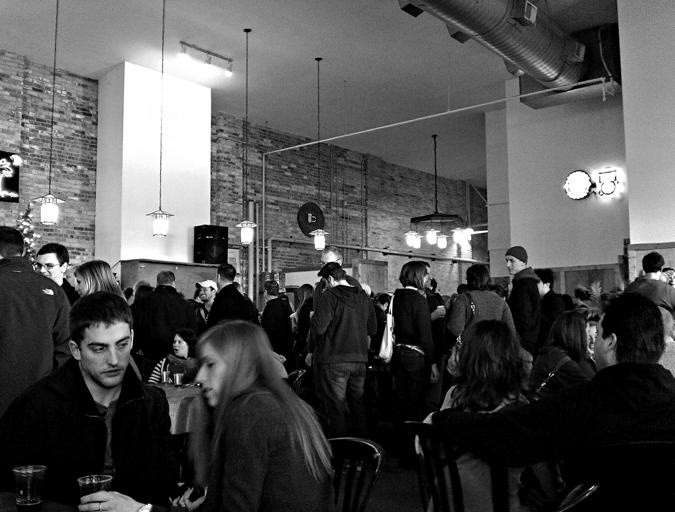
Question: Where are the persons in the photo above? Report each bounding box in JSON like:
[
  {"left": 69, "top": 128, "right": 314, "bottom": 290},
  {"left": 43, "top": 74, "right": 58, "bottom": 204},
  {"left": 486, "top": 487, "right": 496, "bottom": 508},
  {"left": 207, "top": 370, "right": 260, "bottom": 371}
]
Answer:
[
  {"left": 419, "top": 261, "right": 447, "bottom": 412},
  {"left": 662, "top": 268, "right": 675, "bottom": 286},
  {"left": 443, "top": 263, "right": 517, "bottom": 348},
  {"left": 573, "top": 288, "right": 587, "bottom": 309},
  {"left": 312, "top": 245, "right": 343, "bottom": 305},
  {"left": 376, "top": 292, "right": 394, "bottom": 363},
  {"left": 0, "top": 290, "right": 173, "bottom": 512},
  {"left": 147, "top": 329, "right": 202, "bottom": 388},
  {"left": 393, "top": 261, "right": 440, "bottom": 415},
  {"left": 306, "top": 261, "right": 394, "bottom": 434},
  {"left": 622, "top": 251, "right": 675, "bottom": 315},
  {"left": 0, "top": 225, "right": 71, "bottom": 415},
  {"left": 505, "top": 245, "right": 542, "bottom": 355},
  {"left": 195, "top": 280, "right": 218, "bottom": 324},
  {"left": 130, "top": 280, "right": 151, "bottom": 304},
  {"left": 74, "top": 259, "right": 144, "bottom": 389},
  {"left": 35, "top": 243, "right": 81, "bottom": 306},
  {"left": 528, "top": 311, "right": 587, "bottom": 393},
  {"left": 230, "top": 280, "right": 248, "bottom": 301},
  {"left": 535, "top": 267, "right": 563, "bottom": 348},
  {"left": 130, "top": 285, "right": 154, "bottom": 356},
  {"left": 123, "top": 287, "right": 134, "bottom": 305},
  {"left": 584, "top": 320, "right": 599, "bottom": 360},
  {"left": 147, "top": 270, "right": 193, "bottom": 347},
  {"left": 423, "top": 291, "right": 675, "bottom": 512},
  {"left": 207, "top": 263, "right": 254, "bottom": 328},
  {"left": 414, "top": 319, "right": 561, "bottom": 512},
  {"left": 79, "top": 318, "right": 333, "bottom": 512},
  {"left": 260, "top": 280, "right": 314, "bottom": 372}
]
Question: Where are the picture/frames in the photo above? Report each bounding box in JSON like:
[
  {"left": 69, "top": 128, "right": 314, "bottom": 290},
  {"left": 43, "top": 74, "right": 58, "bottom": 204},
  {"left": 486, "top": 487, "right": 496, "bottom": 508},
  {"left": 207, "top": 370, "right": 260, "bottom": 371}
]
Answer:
[{"left": 1, "top": 150, "right": 22, "bottom": 204}]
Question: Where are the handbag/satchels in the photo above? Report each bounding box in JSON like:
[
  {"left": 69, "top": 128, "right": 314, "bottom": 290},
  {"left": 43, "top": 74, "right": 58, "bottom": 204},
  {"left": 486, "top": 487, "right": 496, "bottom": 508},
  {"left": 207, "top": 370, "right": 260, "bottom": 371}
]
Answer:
[
  {"left": 447, "top": 293, "right": 476, "bottom": 379},
  {"left": 379, "top": 296, "right": 395, "bottom": 363}
]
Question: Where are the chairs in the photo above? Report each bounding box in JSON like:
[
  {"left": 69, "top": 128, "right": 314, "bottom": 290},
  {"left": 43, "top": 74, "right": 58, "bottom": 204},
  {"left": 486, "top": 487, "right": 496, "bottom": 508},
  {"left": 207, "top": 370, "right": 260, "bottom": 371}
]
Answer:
[
  {"left": 402, "top": 418, "right": 465, "bottom": 511},
  {"left": 327, "top": 435, "right": 386, "bottom": 512}
]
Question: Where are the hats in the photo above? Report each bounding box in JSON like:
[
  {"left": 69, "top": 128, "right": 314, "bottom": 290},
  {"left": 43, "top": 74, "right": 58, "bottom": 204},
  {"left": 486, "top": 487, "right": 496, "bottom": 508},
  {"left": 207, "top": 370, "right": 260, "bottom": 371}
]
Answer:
[
  {"left": 505, "top": 246, "right": 527, "bottom": 264},
  {"left": 196, "top": 280, "right": 218, "bottom": 291}
]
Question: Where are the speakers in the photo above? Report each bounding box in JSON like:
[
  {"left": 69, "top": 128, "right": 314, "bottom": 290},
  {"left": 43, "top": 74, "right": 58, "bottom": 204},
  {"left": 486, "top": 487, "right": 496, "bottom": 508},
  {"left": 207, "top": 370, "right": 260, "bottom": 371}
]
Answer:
[{"left": 194, "top": 225, "right": 229, "bottom": 265}]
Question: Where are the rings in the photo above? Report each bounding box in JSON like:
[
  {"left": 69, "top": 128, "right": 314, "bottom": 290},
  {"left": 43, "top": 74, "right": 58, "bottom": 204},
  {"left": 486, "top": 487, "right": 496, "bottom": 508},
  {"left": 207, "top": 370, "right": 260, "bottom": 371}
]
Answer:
[{"left": 98, "top": 502, "right": 102, "bottom": 510}]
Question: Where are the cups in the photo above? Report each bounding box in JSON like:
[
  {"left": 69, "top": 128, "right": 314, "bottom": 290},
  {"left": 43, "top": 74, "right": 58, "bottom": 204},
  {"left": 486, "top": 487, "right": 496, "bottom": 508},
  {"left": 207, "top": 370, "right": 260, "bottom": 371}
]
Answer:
[
  {"left": 78, "top": 475, "right": 113, "bottom": 496},
  {"left": 174, "top": 373, "right": 184, "bottom": 387},
  {"left": 13, "top": 464, "right": 46, "bottom": 506},
  {"left": 437, "top": 306, "right": 444, "bottom": 313},
  {"left": 162, "top": 371, "right": 171, "bottom": 384}
]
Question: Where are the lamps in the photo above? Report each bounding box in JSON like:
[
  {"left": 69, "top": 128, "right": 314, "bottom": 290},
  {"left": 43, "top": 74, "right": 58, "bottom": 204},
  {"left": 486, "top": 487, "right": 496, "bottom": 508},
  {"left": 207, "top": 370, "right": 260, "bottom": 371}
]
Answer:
[
  {"left": 309, "top": 56, "right": 328, "bottom": 249},
  {"left": 322, "top": 144, "right": 343, "bottom": 264},
  {"left": 405, "top": 134, "right": 474, "bottom": 250},
  {"left": 33, "top": 1, "right": 65, "bottom": 227},
  {"left": 561, "top": 169, "right": 598, "bottom": 200},
  {"left": 236, "top": 27, "right": 258, "bottom": 247},
  {"left": 146, "top": 1, "right": 175, "bottom": 238}
]
